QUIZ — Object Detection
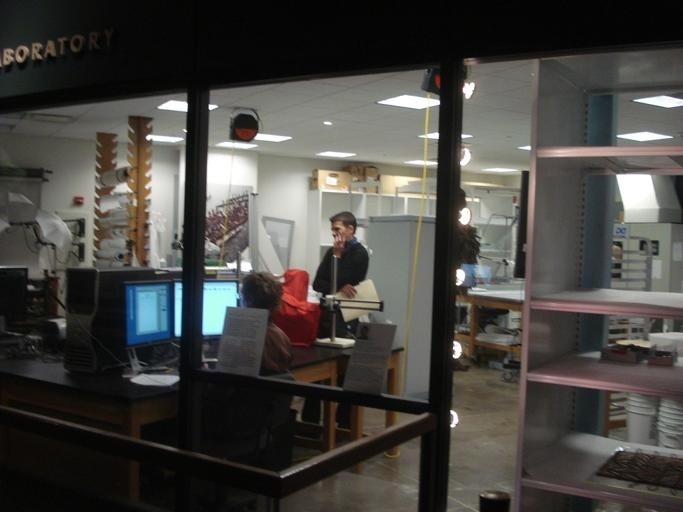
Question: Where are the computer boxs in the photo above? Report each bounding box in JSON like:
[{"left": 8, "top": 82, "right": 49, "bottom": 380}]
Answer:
[{"left": 63, "top": 270, "right": 155, "bottom": 373}]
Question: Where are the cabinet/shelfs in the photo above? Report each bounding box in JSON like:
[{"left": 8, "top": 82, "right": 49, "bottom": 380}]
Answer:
[{"left": 511, "top": 49, "right": 683, "bottom": 512}]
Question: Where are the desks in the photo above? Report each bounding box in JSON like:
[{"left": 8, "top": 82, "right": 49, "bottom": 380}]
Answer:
[
  {"left": 294, "top": 336, "right": 405, "bottom": 459},
  {"left": 0, "top": 346, "right": 344, "bottom": 512}
]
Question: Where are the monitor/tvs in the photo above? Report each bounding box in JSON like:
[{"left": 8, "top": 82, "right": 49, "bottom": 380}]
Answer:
[
  {"left": 172, "top": 278, "right": 242, "bottom": 362},
  {"left": 0, "top": 267, "right": 29, "bottom": 338},
  {"left": 124, "top": 280, "right": 174, "bottom": 376}
]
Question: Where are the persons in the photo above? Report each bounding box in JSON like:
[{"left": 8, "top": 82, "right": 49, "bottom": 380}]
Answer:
[
  {"left": 192, "top": 271, "right": 294, "bottom": 498},
  {"left": 299, "top": 211, "right": 370, "bottom": 430}
]
